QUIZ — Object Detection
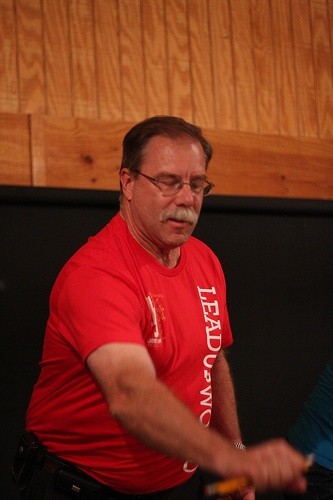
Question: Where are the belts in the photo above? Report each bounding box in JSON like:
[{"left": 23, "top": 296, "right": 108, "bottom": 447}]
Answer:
[{"left": 105, "top": 471, "right": 199, "bottom": 500}]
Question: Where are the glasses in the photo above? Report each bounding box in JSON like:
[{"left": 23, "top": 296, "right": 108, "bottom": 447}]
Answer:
[{"left": 128, "top": 168, "right": 215, "bottom": 198}]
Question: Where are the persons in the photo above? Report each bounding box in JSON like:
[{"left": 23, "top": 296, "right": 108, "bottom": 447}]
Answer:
[
  {"left": 271, "top": 351, "right": 333, "bottom": 500},
  {"left": 0, "top": 113, "right": 312, "bottom": 500}
]
